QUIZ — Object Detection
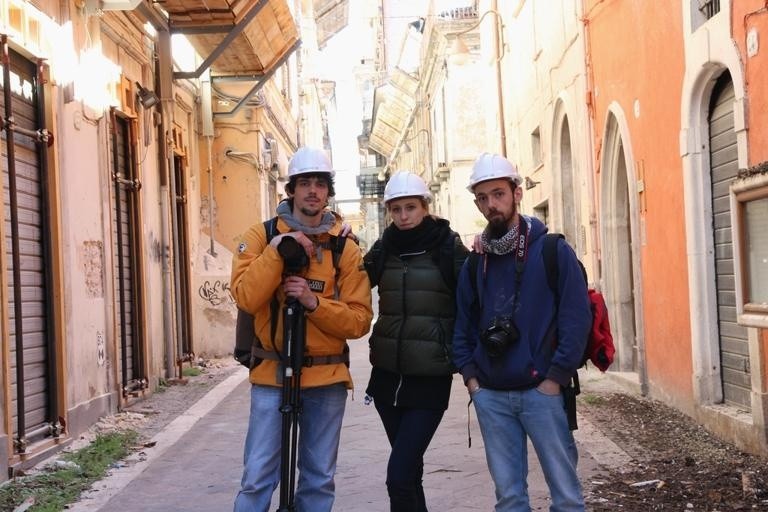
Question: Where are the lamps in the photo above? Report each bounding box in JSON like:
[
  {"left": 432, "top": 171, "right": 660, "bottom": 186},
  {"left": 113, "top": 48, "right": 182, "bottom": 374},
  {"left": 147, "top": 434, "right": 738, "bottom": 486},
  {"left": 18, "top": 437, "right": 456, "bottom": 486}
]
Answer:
[
  {"left": 136, "top": 81, "right": 159, "bottom": 110},
  {"left": 525, "top": 176, "right": 540, "bottom": 190},
  {"left": 408, "top": 18, "right": 425, "bottom": 34}
]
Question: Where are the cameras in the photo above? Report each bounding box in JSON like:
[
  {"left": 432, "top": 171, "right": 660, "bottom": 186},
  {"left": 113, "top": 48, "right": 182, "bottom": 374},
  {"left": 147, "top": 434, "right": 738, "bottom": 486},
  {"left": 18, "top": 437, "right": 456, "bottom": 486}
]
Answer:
[
  {"left": 479, "top": 314, "right": 519, "bottom": 358},
  {"left": 276, "top": 235, "right": 308, "bottom": 276}
]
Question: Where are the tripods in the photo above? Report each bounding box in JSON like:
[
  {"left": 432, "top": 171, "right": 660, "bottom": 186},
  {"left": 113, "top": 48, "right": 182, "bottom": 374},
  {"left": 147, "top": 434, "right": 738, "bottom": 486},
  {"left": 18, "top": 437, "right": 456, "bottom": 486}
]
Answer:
[{"left": 278, "top": 296, "right": 306, "bottom": 512}]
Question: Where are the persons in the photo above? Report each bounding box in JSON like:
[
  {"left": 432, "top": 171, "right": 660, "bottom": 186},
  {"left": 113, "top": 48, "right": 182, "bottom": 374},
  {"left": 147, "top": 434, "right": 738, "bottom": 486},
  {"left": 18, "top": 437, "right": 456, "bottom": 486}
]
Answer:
[
  {"left": 341, "top": 173, "right": 486, "bottom": 510},
  {"left": 451, "top": 152, "right": 594, "bottom": 511},
  {"left": 232, "top": 144, "right": 376, "bottom": 510}
]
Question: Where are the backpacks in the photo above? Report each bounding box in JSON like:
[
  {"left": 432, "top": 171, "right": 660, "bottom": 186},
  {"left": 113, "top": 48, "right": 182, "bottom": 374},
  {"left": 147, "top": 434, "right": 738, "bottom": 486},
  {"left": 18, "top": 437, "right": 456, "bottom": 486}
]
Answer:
[
  {"left": 468, "top": 234, "right": 615, "bottom": 373},
  {"left": 234, "top": 216, "right": 348, "bottom": 369}
]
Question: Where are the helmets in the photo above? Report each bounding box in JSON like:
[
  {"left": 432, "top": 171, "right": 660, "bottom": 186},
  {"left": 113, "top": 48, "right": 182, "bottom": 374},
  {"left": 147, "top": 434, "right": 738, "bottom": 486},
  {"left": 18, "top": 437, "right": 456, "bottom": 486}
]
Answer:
[
  {"left": 466, "top": 152, "right": 523, "bottom": 192},
  {"left": 384, "top": 170, "right": 433, "bottom": 208},
  {"left": 288, "top": 146, "right": 336, "bottom": 178}
]
